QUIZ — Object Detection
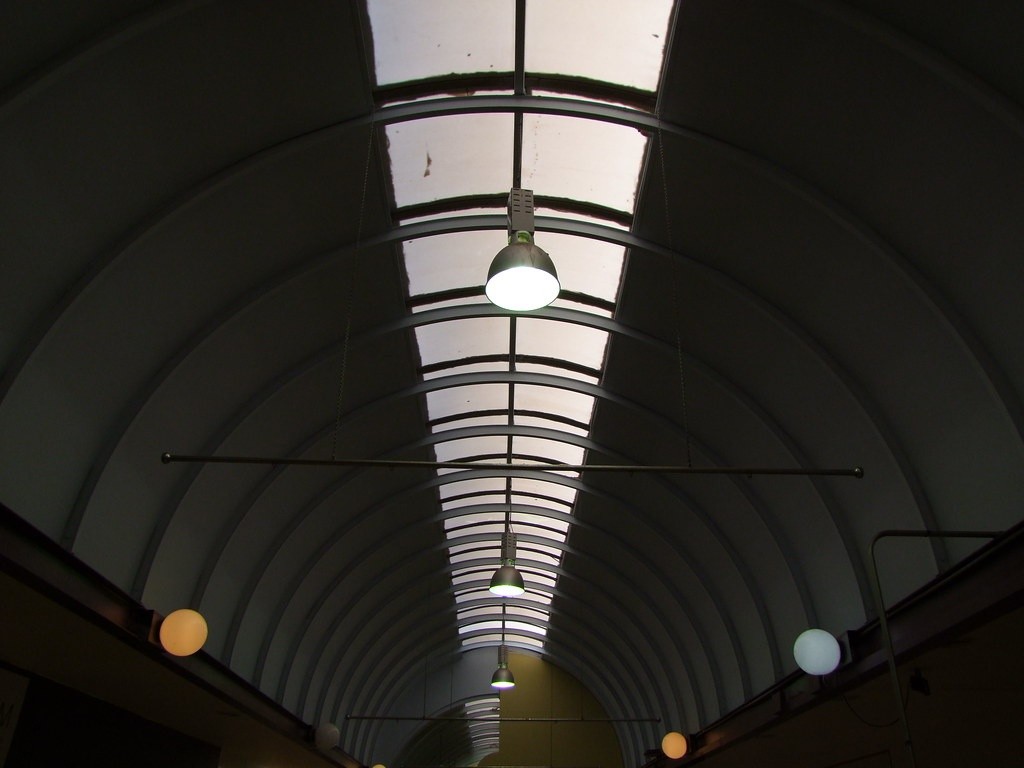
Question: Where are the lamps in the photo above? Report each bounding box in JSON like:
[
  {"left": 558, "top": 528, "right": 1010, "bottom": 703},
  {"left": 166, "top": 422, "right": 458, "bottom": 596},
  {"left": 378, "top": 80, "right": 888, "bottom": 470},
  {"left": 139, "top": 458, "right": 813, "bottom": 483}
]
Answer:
[
  {"left": 793, "top": 628, "right": 865, "bottom": 675},
  {"left": 490, "top": 645, "right": 515, "bottom": 688},
  {"left": 484, "top": 187, "right": 561, "bottom": 313},
  {"left": 358, "top": 764, "right": 386, "bottom": 768},
  {"left": 137, "top": 609, "right": 208, "bottom": 657},
  {"left": 488, "top": 528, "right": 525, "bottom": 597},
  {"left": 300, "top": 723, "right": 340, "bottom": 752},
  {"left": 662, "top": 732, "right": 705, "bottom": 759}
]
